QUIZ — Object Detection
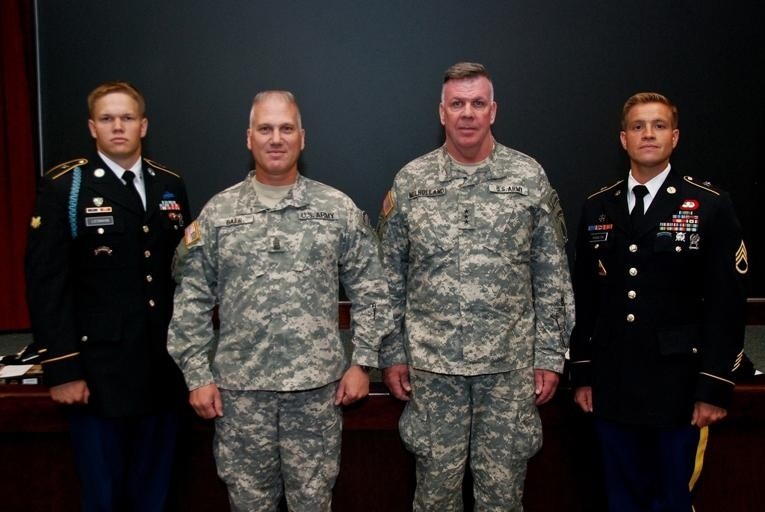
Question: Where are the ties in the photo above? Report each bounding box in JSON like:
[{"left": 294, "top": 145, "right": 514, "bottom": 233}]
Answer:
[
  {"left": 630, "top": 185, "right": 649, "bottom": 223},
  {"left": 123, "top": 171, "right": 144, "bottom": 213}
]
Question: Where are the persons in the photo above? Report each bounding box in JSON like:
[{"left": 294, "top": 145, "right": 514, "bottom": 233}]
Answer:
[
  {"left": 166, "top": 86, "right": 395, "bottom": 511},
  {"left": 572, "top": 92, "right": 751, "bottom": 507},
  {"left": 377, "top": 61, "right": 580, "bottom": 512},
  {"left": 28, "top": 79, "right": 208, "bottom": 512}
]
